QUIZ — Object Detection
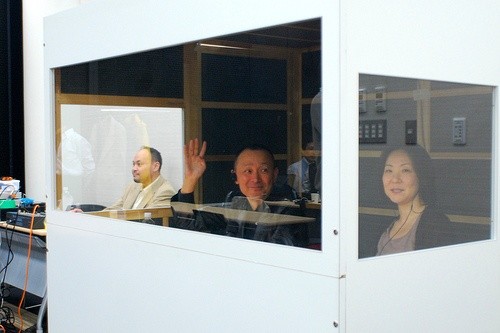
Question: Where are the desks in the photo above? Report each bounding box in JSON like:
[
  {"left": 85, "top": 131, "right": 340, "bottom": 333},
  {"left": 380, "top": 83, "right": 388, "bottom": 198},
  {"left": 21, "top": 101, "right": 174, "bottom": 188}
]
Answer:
[
  {"left": 170, "top": 201, "right": 316, "bottom": 249},
  {"left": 0, "top": 212, "right": 48, "bottom": 333}
]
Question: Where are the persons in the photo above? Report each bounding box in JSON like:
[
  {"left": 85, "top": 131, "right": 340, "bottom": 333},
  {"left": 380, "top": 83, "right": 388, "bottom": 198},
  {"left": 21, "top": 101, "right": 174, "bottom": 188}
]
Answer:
[
  {"left": 67, "top": 147, "right": 177, "bottom": 212},
  {"left": 288, "top": 142, "right": 315, "bottom": 199},
  {"left": 359, "top": 144, "right": 453, "bottom": 259},
  {"left": 60, "top": 117, "right": 94, "bottom": 205},
  {"left": 311, "top": 86, "right": 321, "bottom": 204},
  {"left": 171, "top": 138, "right": 308, "bottom": 248}
]
